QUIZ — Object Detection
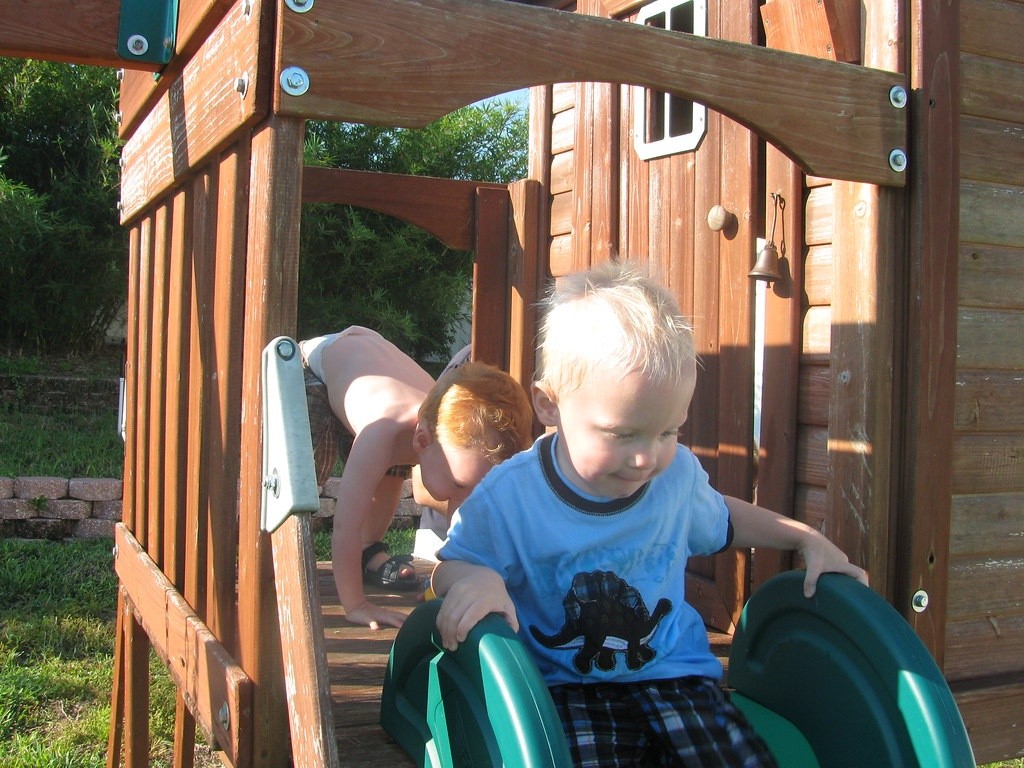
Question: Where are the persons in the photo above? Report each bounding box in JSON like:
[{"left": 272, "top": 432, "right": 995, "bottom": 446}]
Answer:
[
  {"left": 298, "top": 325, "right": 533, "bottom": 631},
  {"left": 412, "top": 342, "right": 471, "bottom": 561},
  {"left": 429, "top": 264, "right": 868, "bottom": 768}
]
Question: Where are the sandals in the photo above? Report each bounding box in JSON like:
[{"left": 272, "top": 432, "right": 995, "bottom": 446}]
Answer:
[{"left": 361, "top": 542, "right": 421, "bottom": 589}]
